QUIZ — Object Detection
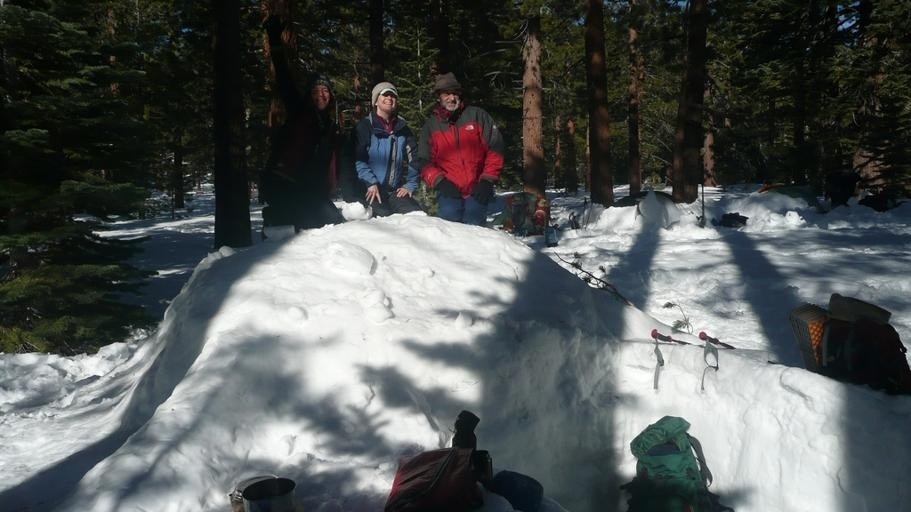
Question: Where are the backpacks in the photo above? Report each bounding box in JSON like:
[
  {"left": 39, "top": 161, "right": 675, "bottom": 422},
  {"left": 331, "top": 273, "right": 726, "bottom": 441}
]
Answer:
[
  {"left": 502, "top": 191, "right": 550, "bottom": 235},
  {"left": 821, "top": 293, "right": 910, "bottom": 397},
  {"left": 620, "top": 415, "right": 733, "bottom": 511}
]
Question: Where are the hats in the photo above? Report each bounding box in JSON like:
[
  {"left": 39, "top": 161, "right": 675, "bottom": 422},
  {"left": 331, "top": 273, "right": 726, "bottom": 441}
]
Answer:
[
  {"left": 306, "top": 71, "right": 337, "bottom": 101},
  {"left": 371, "top": 81, "right": 398, "bottom": 109},
  {"left": 435, "top": 72, "right": 464, "bottom": 98}
]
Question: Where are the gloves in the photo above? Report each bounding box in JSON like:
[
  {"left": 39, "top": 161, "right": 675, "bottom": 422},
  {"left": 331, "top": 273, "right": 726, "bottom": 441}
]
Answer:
[
  {"left": 471, "top": 178, "right": 496, "bottom": 207},
  {"left": 438, "top": 178, "right": 463, "bottom": 198}
]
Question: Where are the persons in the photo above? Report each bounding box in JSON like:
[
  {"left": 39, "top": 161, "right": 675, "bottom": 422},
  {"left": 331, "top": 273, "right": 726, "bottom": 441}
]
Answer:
[
  {"left": 413, "top": 72, "right": 506, "bottom": 227},
  {"left": 262, "top": 32, "right": 344, "bottom": 232},
  {"left": 352, "top": 82, "right": 422, "bottom": 218}
]
